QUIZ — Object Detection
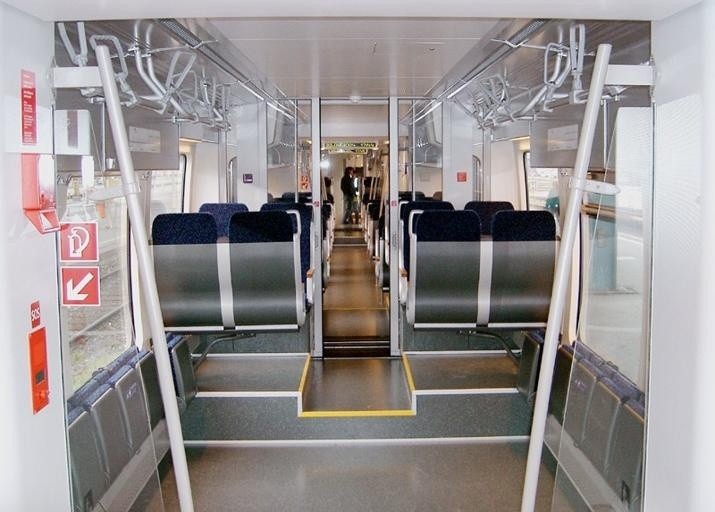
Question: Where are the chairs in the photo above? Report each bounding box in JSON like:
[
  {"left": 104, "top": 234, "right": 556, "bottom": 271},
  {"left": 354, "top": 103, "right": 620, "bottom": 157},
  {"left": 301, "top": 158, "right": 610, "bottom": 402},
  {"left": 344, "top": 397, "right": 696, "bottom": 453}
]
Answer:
[
  {"left": 404, "top": 205, "right": 483, "bottom": 334},
  {"left": 225, "top": 208, "right": 309, "bottom": 330},
  {"left": 196, "top": 182, "right": 334, "bottom": 286},
  {"left": 486, "top": 205, "right": 557, "bottom": 329},
  {"left": 360, "top": 183, "right": 516, "bottom": 302},
  {"left": 149, "top": 209, "right": 225, "bottom": 332}
]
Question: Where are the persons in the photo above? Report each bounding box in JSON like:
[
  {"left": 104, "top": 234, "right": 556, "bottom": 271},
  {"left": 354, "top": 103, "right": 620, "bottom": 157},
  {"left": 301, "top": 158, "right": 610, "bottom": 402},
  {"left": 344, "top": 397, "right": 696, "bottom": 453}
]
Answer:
[
  {"left": 341, "top": 166, "right": 356, "bottom": 224},
  {"left": 349, "top": 169, "right": 361, "bottom": 225}
]
